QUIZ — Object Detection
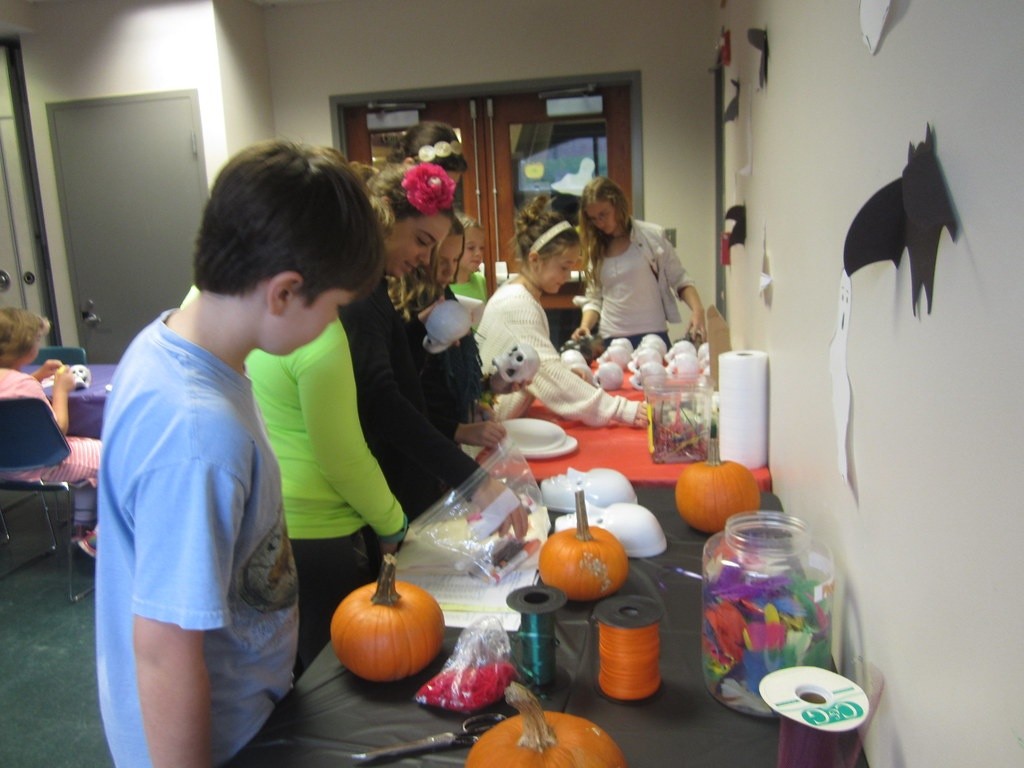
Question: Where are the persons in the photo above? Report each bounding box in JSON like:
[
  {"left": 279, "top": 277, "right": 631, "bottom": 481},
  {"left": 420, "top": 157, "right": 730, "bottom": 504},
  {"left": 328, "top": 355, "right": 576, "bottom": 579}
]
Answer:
[
  {"left": 247, "top": 320, "right": 404, "bottom": 677},
  {"left": 475, "top": 195, "right": 650, "bottom": 426},
  {"left": 95, "top": 142, "right": 385, "bottom": 768},
  {"left": 571, "top": 177, "right": 707, "bottom": 353},
  {"left": 345, "top": 124, "right": 533, "bottom": 539},
  {"left": 0, "top": 308, "right": 100, "bottom": 557}
]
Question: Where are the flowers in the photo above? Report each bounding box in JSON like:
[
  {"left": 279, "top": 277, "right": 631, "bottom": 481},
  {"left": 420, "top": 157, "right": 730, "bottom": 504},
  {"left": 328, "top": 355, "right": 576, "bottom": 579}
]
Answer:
[{"left": 401, "top": 164, "right": 457, "bottom": 216}]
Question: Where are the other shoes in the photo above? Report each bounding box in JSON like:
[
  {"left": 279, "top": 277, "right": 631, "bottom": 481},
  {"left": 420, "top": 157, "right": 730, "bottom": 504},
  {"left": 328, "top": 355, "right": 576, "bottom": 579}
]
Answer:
[
  {"left": 77, "top": 530, "right": 98, "bottom": 559},
  {"left": 71, "top": 525, "right": 91, "bottom": 542}
]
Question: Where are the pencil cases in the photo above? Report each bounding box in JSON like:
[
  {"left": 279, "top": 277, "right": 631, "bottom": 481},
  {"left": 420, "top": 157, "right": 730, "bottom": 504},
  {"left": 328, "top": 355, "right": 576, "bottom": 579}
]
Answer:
[{"left": 409, "top": 433, "right": 552, "bottom": 585}]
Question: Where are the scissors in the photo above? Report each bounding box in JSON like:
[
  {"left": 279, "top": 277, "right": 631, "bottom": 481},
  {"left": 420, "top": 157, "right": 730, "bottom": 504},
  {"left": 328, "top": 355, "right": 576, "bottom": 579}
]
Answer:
[{"left": 351, "top": 713, "right": 508, "bottom": 764}]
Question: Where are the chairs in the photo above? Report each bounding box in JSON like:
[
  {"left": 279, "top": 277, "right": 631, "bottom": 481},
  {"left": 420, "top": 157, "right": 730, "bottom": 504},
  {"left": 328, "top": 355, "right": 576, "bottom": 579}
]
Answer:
[
  {"left": 0, "top": 397, "right": 94, "bottom": 603},
  {"left": 31, "top": 347, "right": 86, "bottom": 366}
]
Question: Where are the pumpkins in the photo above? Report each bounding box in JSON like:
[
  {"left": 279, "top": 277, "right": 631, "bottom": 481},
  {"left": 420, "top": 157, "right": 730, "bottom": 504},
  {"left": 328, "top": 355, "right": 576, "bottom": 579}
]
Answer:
[
  {"left": 675, "top": 438, "right": 762, "bottom": 533},
  {"left": 538, "top": 489, "right": 627, "bottom": 601},
  {"left": 464, "top": 680, "right": 628, "bottom": 768},
  {"left": 330, "top": 552, "right": 445, "bottom": 682}
]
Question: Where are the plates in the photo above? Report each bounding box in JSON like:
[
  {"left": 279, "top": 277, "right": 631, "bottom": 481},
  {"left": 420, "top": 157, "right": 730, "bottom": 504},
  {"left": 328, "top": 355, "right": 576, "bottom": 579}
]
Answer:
[
  {"left": 499, "top": 418, "right": 566, "bottom": 451},
  {"left": 521, "top": 435, "right": 578, "bottom": 459}
]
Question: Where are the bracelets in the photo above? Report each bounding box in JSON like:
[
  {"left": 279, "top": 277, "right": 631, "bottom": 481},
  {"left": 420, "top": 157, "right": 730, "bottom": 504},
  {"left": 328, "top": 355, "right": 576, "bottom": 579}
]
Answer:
[{"left": 384, "top": 518, "right": 408, "bottom": 542}]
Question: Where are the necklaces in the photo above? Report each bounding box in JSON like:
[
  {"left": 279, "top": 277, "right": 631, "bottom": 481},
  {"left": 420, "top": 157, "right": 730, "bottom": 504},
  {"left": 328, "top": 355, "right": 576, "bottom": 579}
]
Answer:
[{"left": 614, "top": 235, "right": 623, "bottom": 239}]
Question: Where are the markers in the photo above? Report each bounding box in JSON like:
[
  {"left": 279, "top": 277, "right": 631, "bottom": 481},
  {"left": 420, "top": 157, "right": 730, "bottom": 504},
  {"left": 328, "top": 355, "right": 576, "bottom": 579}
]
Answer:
[{"left": 454, "top": 494, "right": 542, "bottom": 585}]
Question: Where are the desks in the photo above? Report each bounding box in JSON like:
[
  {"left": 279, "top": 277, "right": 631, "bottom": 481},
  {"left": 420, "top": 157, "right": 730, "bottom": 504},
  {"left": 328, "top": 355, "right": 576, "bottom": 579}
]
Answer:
[
  {"left": 223, "top": 360, "right": 870, "bottom": 768},
  {"left": 19, "top": 365, "right": 117, "bottom": 441}
]
